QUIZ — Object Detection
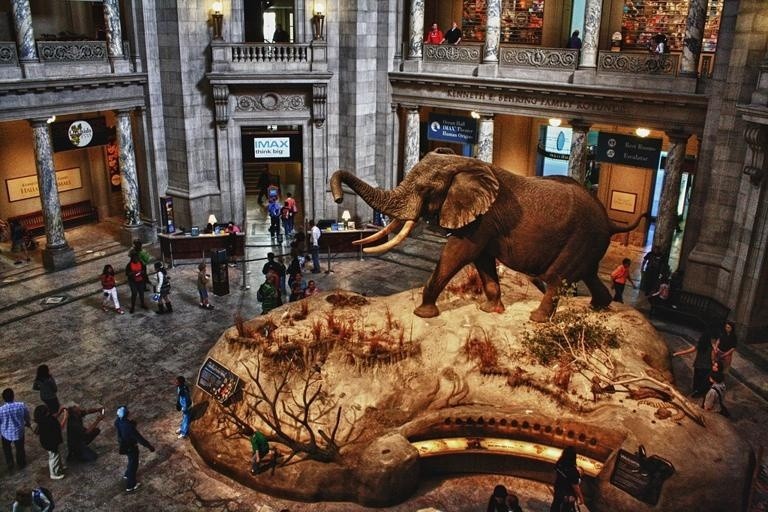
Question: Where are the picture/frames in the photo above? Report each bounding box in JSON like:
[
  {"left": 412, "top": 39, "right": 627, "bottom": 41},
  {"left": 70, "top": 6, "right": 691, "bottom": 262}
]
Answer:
[
  {"left": 5, "top": 167, "right": 83, "bottom": 202},
  {"left": 609, "top": 190, "right": 638, "bottom": 214}
]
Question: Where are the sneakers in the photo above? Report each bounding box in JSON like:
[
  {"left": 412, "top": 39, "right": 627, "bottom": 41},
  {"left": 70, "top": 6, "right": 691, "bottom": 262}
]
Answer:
[
  {"left": 175, "top": 429, "right": 185, "bottom": 439},
  {"left": 51, "top": 474, "right": 64, "bottom": 479}
]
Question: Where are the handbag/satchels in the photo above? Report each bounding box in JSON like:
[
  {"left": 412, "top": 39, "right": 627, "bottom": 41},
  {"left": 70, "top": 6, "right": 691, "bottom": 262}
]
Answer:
[{"left": 720, "top": 405, "right": 729, "bottom": 417}]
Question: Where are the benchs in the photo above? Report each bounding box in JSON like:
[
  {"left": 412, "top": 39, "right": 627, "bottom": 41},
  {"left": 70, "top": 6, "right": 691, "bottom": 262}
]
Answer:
[
  {"left": 651, "top": 289, "right": 732, "bottom": 336},
  {"left": 7, "top": 198, "right": 99, "bottom": 232}
]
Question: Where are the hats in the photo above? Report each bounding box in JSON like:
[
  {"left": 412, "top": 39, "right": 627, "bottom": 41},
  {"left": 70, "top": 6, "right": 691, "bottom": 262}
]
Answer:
[{"left": 117, "top": 407, "right": 128, "bottom": 419}]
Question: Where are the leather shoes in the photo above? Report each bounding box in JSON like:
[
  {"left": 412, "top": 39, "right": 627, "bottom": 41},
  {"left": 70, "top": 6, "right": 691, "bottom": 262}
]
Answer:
[{"left": 126, "top": 483, "right": 141, "bottom": 492}]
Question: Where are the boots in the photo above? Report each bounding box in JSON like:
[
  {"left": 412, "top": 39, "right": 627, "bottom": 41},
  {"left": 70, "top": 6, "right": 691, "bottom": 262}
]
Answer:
[{"left": 156, "top": 302, "right": 173, "bottom": 313}]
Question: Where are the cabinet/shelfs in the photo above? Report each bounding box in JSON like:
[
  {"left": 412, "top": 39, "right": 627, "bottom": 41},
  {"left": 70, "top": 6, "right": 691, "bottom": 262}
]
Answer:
[
  {"left": 621, "top": 0, "right": 724, "bottom": 53},
  {"left": 461, "top": 0, "right": 544, "bottom": 45}
]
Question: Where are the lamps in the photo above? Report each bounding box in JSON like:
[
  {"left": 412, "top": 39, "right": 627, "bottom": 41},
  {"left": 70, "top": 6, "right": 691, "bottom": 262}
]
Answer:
[
  {"left": 313, "top": 3, "right": 326, "bottom": 41},
  {"left": 211, "top": 1, "right": 223, "bottom": 39},
  {"left": 341, "top": 210, "right": 351, "bottom": 228},
  {"left": 208, "top": 214, "right": 218, "bottom": 232}
]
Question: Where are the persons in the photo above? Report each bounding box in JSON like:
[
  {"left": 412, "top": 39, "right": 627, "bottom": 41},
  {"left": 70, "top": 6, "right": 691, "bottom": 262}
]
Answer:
[
  {"left": 242, "top": 425, "right": 270, "bottom": 476},
  {"left": 273, "top": 24, "right": 289, "bottom": 42},
  {"left": 173, "top": 375, "right": 191, "bottom": 439},
  {"left": 672, "top": 331, "right": 717, "bottom": 394},
  {"left": 423, "top": 21, "right": 443, "bottom": 46},
  {"left": 610, "top": 257, "right": 637, "bottom": 304},
  {"left": 66, "top": 400, "right": 105, "bottom": 464},
  {"left": 22, "top": 227, "right": 40, "bottom": 251},
  {"left": 502, "top": 493, "right": 521, "bottom": 511},
  {"left": 640, "top": 245, "right": 674, "bottom": 312},
  {"left": 113, "top": 405, "right": 156, "bottom": 492},
  {"left": 714, "top": 321, "right": 738, "bottom": 390},
  {"left": 13, "top": 486, "right": 53, "bottom": 512},
  {"left": 568, "top": 30, "right": 581, "bottom": 50},
  {"left": 0, "top": 387, "right": 33, "bottom": 468},
  {"left": 692, "top": 360, "right": 722, "bottom": 399},
  {"left": 704, "top": 373, "right": 726, "bottom": 413},
  {"left": 8, "top": 216, "right": 34, "bottom": 265},
  {"left": 97, "top": 160, "right": 322, "bottom": 320},
  {"left": 441, "top": 23, "right": 463, "bottom": 45},
  {"left": 547, "top": 444, "right": 586, "bottom": 511},
  {"left": 487, "top": 483, "right": 508, "bottom": 510},
  {"left": 33, "top": 364, "right": 60, "bottom": 411},
  {"left": 31, "top": 403, "right": 68, "bottom": 480}
]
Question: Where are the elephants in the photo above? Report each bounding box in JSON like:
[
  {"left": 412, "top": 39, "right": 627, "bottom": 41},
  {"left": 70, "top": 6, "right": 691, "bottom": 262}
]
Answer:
[{"left": 330, "top": 147, "right": 652, "bottom": 323}]
